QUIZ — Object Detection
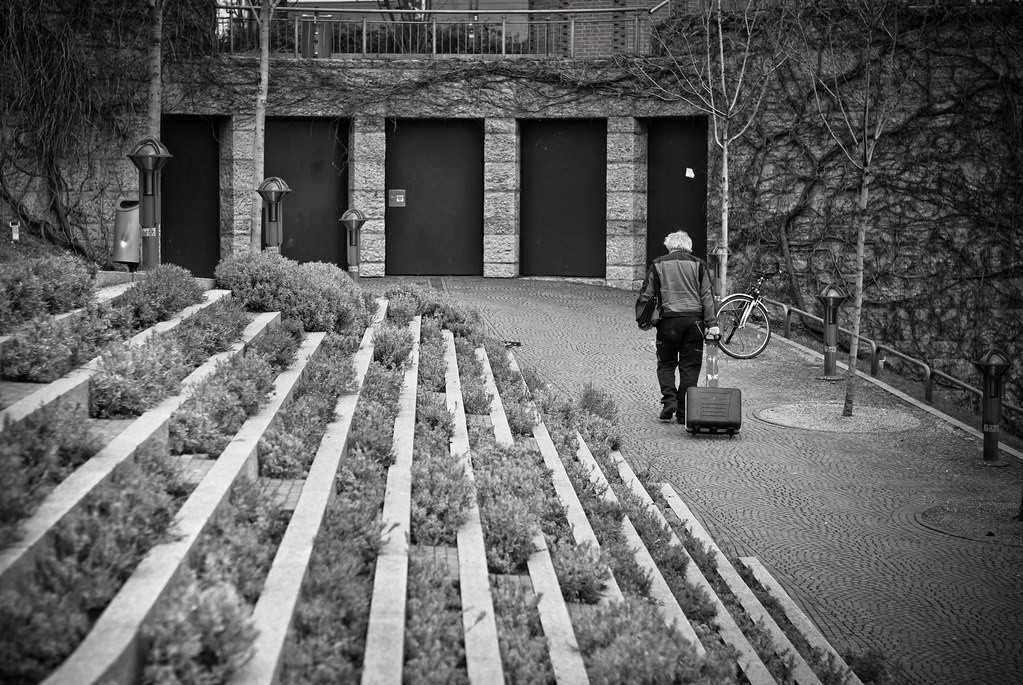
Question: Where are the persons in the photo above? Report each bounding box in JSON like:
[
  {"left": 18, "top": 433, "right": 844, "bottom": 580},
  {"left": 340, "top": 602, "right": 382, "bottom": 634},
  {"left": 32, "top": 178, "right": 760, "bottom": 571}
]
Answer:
[{"left": 635, "top": 229, "right": 720, "bottom": 426}]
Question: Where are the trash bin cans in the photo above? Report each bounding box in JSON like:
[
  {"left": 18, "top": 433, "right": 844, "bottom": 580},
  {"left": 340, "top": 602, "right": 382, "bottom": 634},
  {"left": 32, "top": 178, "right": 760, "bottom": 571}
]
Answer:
[
  {"left": 301, "top": 14, "right": 333, "bottom": 59},
  {"left": 112, "top": 198, "right": 142, "bottom": 272}
]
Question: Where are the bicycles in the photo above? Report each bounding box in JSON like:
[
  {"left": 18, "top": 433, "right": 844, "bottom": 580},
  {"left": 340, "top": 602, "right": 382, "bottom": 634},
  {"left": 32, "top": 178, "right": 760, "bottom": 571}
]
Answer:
[{"left": 714, "top": 269, "right": 784, "bottom": 360}]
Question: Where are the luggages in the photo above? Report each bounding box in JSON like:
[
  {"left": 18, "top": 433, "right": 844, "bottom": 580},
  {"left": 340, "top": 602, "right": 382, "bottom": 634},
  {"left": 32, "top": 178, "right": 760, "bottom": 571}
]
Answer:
[{"left": 685, "top": 334, "right": 741, "bottom": 438}]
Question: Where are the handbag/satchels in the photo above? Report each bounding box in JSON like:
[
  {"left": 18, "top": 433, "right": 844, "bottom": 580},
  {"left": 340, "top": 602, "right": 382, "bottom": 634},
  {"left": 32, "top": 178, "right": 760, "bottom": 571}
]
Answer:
[{"left": 640, "top": 296, "right": 661, "bottom": 328}]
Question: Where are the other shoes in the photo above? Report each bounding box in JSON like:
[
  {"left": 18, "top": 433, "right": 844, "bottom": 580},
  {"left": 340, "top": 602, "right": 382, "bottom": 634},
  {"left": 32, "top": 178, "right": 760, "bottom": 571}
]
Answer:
[
  {"left": 676, "top": 417, "right": 685, "bottom": 425},
  {"left": 660, "top": 403, "right": 677, "bottom": 420}
]
